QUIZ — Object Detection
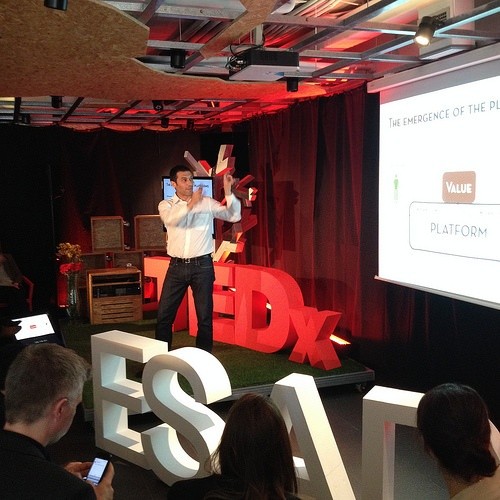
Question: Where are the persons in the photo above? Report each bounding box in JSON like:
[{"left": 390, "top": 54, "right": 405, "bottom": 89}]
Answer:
[
  {"left": 135, "top": 164, "right": 241, "bottom": 378},
  {"left": 0, "top": 342, "right": 115, "bottom": 500},
  {"left": 416, "top": 384, "right": 500, "bottom": 500},
  {"left": 0, "top": 252, "right": 32, "bottom": 431},
  {"left": 166, "top": 391, "right": 304, "bottom": 500}
]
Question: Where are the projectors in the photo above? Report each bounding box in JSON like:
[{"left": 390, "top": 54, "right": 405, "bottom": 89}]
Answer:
[{"left": 228, "top": 49, "right": 299, "bottom": 82}]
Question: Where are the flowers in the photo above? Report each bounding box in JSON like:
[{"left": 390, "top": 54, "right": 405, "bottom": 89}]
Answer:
[{"left": 56, "top": 243, "right": 83, "bottom": 277}]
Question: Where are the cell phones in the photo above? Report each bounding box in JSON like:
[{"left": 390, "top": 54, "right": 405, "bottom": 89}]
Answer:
[{"left": 85, "top": 451, "right": 113, "bottom": 486}]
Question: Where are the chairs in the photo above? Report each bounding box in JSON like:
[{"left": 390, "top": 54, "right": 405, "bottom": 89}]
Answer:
[{"left": 0, "top": 275, "right": 34, "bottom": 313}]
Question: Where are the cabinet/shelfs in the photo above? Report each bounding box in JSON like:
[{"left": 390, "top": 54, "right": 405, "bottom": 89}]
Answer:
[{"left": 86, "top": 267, "right": 143, "bottom": 324}]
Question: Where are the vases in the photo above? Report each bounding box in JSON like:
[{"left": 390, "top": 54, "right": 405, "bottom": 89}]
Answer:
[{"left": 66, "top": 274, "right": 81, "bottom": 320}]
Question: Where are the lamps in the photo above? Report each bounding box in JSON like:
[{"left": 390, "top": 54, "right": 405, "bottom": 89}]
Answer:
[
  {"left": 44, "top": 0, "right": 67, "bottom": 11},
  {"left": 161, "top": 118, "right": 169, "bottom": 127},
  {"left": 170, "top": 48, "right": 185, "bottom": 68},
  {"left": 52, "top": 96, "right": 62, "bottom": 108},
  {"left": 152, "top": 100, "right": 163, "bottom": 111},
  {"left": 414, "top": 16, "right": 444, "bottom": 47},
  {"left": 187, "top": 119, "right": 194, "bottom": 129},
  {"left": 287, "top": 77, "right": 298, "bottom": 92}
]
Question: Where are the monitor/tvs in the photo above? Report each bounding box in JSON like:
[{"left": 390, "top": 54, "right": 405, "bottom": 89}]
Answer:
[{"left": 160, "top": 176, "right": 216, "bottom": 237}]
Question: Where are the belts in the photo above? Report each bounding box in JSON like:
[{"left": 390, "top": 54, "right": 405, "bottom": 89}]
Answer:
[{"left": 170, "top": 254, "right": 211, "bottom": 264}]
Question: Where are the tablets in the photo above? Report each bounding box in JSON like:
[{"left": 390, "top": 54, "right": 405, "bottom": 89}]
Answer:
[{"left": 7, "top": 312, "right": 58, "bottom": 343}]
